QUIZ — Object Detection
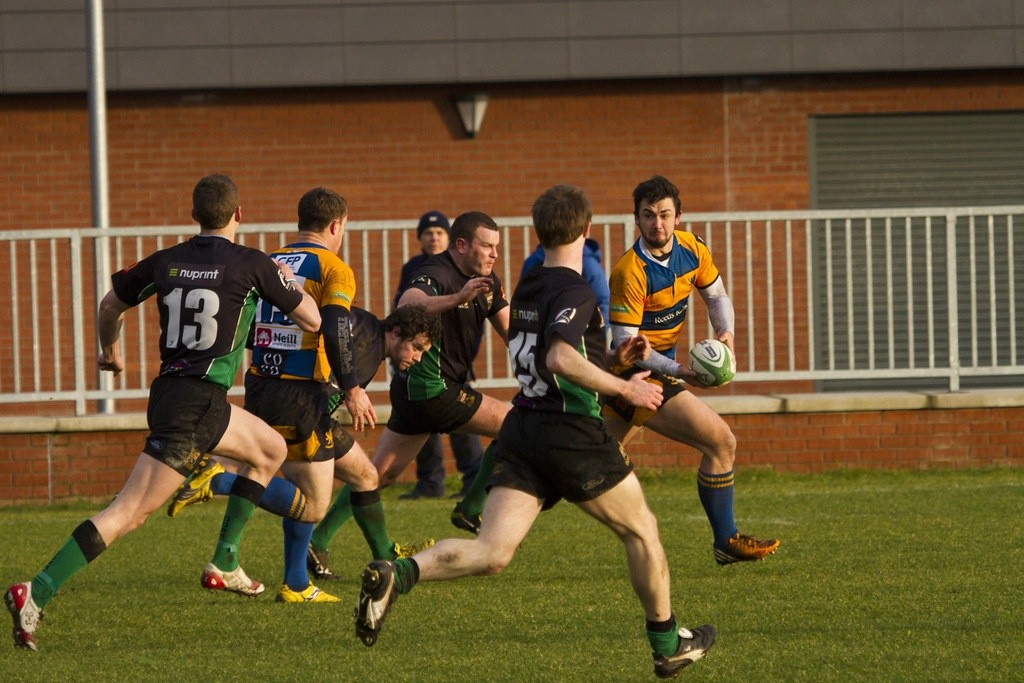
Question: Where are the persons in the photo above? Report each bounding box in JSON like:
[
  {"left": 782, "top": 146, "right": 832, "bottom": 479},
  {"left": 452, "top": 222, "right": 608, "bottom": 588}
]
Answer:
[
  {"left": 358, "top": 185, "right": 714, "bottom": 678},
  {"left": 168, "top": 186, "right": 376, "bottom": 601},
  {"left": 308, "top": 213, "right": 509, "bottom": 580},
  {"left": 394, "top": 211, "right": 482, "bottom": 499},
  {"left": 521, "top": 176, "right": 780, "bottom": 565},
  {"left": 243, "top": 303, "right": 441, "bottom": 560},
  {"left": 5, "top": 173, "right": 322, "bottom": 650}
]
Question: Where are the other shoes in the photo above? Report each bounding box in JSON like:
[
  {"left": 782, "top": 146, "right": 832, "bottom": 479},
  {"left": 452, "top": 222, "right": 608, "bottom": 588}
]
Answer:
[
  {"left": 397, "top": 489, "right": 443, "bottom": 499},
  {"left": 449, "top": 488, "right": 471, "bottom": 498}
]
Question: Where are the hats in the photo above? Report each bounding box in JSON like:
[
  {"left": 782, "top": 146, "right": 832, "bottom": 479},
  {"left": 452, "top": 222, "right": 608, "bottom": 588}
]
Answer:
[{"left": 416, "top": 212, "right": 450, "bottom": 239}]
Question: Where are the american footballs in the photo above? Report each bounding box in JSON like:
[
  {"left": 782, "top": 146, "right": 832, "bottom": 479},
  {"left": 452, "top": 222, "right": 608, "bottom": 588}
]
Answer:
[{"left": 690, "top": 338, "right": 738, "bottom": 387}]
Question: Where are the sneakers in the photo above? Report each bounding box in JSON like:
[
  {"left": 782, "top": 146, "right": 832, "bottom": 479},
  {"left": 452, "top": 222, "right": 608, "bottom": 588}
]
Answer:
[
  {"left": 200, "top": 562, "right": 265, "bottom": 595},
  {"left": 4, "top": 580, "right": 43, "bottom": 653},
  {"left": 166, "top": 455, "right": 224, "bottom": 518},
  {"left": 276, "top": 580, "right": 342, "bottom": 603},
  {"left": 354, "top": 560, "right": 397, "bottom": 647},
  {"left": 713, "top": 530, "right": 780, "bottom": 566},
  {"left": 653, "top": 624, "right": 717, "bottom": 677},
  {"left": 451, "top": 502, "right": 480, "bottom": 534},
  {"left": 307, "top": 544, "right": 340, "bottom": 584}
]
